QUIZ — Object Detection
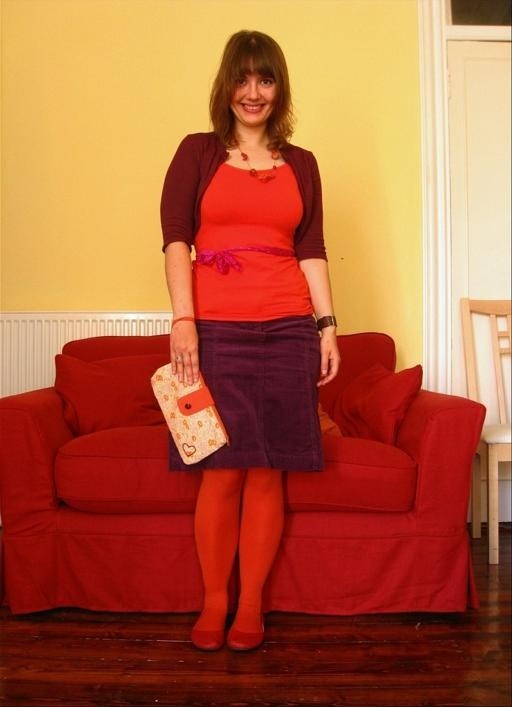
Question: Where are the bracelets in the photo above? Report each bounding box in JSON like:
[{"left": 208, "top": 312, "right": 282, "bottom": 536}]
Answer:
[
  {"left": 171, "top": 317, "right": 196, "bottom": 324},
  {"left": 317, "top": 316, "right": 338, "bottom": 330}
]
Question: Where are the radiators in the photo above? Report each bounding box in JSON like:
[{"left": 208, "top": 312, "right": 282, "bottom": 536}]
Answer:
[{"left": 0, "top": 311, "right": 174, "bottom": 400}]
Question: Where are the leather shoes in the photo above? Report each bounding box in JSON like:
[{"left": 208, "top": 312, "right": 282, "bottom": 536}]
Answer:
[
  {"left": 226, "top": 608, "right": 265, "bottom": 651},
  {"left": 193, "top": 612, "right": 226, "bottom": 652}
]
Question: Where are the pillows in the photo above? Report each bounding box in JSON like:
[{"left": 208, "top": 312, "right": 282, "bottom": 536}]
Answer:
[
  {"left": 53, "top": 350, "right": 172, "bottom": 436},
  {"left": 329, "top": 360, "right": 424, "bottom": 445}
]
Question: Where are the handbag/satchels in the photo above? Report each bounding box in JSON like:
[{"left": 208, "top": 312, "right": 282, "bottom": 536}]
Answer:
[{"left": 150, "top": 358, "right": 230, "bottom": 467}]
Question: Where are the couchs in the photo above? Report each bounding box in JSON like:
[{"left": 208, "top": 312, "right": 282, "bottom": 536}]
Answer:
[{"left": 1, "top": 330, "right": 489, "bottom": 616}]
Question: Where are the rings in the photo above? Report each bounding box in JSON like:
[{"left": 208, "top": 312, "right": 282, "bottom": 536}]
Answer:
[{"left": 175, "top": 356, "right": 182, "bottom": 363}]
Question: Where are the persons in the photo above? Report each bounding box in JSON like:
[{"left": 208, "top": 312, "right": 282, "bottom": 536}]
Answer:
[{"left": 159, "top": 29, "right": 342, "bottom": 652}]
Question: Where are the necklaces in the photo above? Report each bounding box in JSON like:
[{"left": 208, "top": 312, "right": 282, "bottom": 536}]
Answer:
[{"left": 230, "top": 136, "right": 279, "bottom": 183}]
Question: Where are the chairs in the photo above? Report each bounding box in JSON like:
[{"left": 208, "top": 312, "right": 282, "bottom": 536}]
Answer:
[{"left": 459, "top": 297, "right": 511, "bottom": 565}]
